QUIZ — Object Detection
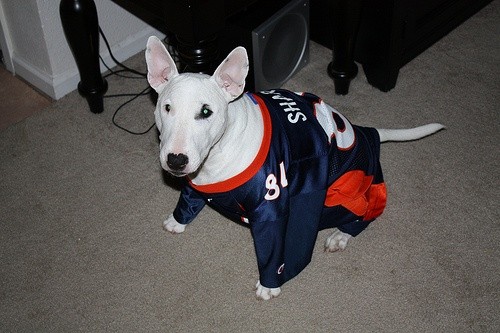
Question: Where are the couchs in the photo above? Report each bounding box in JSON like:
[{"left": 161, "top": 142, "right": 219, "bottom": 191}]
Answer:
[{"left": 308, "top": 0, "right": 494, "bottom": 92}]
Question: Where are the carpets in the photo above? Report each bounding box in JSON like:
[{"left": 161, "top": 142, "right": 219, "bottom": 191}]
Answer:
[{"left": 0, "top": 1, "right": 500, "bottom": 333}]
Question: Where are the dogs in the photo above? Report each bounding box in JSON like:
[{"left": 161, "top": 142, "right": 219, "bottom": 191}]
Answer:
[{"left": 145, "top": 35, "right": 449, "bottom": 302}]
